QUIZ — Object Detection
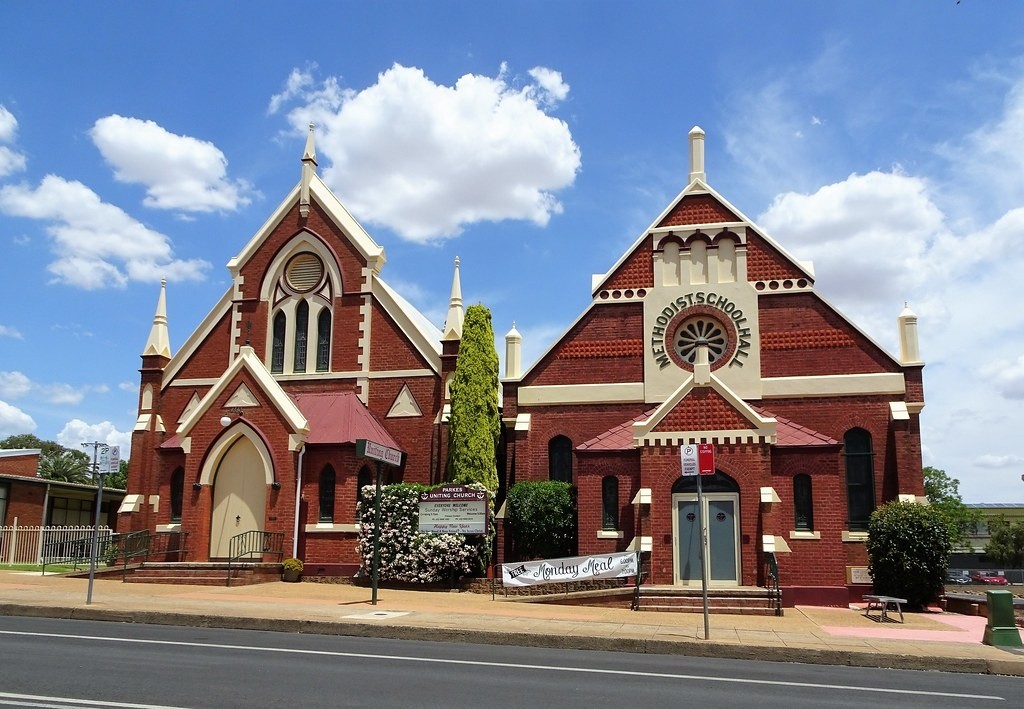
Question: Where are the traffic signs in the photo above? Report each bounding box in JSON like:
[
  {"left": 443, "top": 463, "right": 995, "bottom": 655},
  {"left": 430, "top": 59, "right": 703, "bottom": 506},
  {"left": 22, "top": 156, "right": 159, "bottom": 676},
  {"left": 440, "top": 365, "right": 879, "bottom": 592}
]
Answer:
[
  {"left": 99, "top": 446, "right": 108, "bottom": 474},
  {"left": 109, "top": 446, "right": 121, "bottom": 474}
]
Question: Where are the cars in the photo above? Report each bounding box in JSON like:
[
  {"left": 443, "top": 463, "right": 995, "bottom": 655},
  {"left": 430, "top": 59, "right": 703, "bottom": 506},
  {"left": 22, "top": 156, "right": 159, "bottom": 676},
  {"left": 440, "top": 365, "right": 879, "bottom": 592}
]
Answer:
[
  {"left": 971, "top": 570, "right": 1008, "bottom": 586},
  {"left": 943, "top": 569, "right": 973, "bottom": 585}
]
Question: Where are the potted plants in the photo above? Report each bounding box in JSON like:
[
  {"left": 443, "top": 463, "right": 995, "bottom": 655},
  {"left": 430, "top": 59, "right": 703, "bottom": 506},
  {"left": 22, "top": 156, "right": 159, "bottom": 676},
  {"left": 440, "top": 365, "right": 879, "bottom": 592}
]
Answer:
[
  {"left": 105, "top": 544, "right": 119, "bottom": 567},
  {"left": 281, "top": 557, "right": 304, "bottom": 582}
]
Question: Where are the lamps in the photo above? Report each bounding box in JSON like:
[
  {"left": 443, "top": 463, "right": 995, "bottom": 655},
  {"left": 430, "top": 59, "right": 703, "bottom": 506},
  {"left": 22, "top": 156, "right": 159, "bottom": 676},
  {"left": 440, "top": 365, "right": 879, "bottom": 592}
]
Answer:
[{"left": 220, "top": 410, "right": 244, "bottom": 427}]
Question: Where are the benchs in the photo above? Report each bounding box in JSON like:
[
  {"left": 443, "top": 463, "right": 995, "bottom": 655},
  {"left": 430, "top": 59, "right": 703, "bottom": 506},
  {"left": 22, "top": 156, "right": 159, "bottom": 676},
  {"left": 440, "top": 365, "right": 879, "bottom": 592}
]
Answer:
[{"left": 862, "top": 595, "right": 908, "bottom": 622}]
